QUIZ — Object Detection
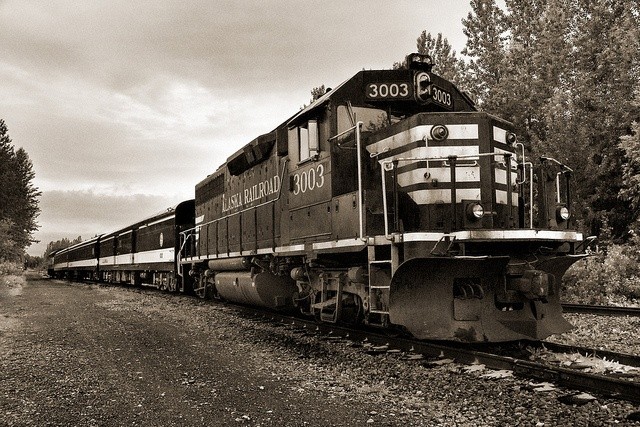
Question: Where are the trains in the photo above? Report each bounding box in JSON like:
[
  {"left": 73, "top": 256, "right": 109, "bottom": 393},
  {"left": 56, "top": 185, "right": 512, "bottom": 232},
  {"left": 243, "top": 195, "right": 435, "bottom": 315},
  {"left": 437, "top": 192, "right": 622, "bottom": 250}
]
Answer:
[{"left": 47, "top": 54, "right": 597, "bottom": 343}]
